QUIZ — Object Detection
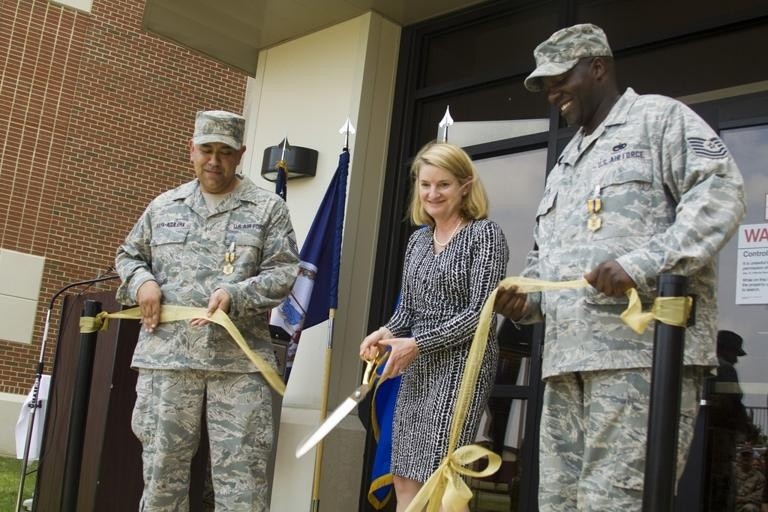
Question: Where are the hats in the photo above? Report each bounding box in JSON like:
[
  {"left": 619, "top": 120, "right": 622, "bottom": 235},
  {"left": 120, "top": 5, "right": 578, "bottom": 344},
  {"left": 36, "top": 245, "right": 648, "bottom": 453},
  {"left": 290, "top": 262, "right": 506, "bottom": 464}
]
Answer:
[
  {"left": 524, "top": 22, "right": 613, "bottom": 94},
  {"left": 192, "top": 110, "right": 245, "bottom": 152}
]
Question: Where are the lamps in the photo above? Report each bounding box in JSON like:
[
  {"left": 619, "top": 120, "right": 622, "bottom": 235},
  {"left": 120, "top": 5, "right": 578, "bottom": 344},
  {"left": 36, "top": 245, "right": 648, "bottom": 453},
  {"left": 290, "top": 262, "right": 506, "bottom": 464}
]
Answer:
[{"left": 259, "top": 137, "right": 317, "bottom": 182}]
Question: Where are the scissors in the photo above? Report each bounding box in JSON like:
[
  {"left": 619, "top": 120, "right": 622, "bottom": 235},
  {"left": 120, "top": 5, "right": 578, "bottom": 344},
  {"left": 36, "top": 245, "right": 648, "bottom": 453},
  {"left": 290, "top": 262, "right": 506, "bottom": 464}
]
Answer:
[{"left": 295, "top": 343, "right": 392, "bottom": 460}]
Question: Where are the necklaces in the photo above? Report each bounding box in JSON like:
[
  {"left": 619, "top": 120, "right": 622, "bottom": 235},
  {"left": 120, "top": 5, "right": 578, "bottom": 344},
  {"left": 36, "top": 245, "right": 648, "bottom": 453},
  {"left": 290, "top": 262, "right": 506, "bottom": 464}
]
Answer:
[{"left": 433, "top": 217, "right": 464, "bottom": 248}]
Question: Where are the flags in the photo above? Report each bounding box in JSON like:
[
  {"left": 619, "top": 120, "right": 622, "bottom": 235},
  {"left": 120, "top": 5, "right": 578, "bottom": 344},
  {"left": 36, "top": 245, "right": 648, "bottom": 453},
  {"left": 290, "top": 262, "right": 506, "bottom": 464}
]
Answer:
[{"left": 268, "top": 149, "right": 350, "bottom": 385}]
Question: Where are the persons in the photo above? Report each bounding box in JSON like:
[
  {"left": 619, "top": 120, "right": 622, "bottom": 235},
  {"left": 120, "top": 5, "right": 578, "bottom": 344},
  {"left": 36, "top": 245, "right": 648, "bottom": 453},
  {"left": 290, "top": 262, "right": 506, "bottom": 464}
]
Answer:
[
  {"left": 491, "top": 22, "right": 748, "bottom": 512},
  {"left": 115, "top": 109, "right": 300, "bottom": 512},
  {"left": 357, "top": 141, "right": 510, "bottom": 511},
  {"left": 707, "top": 329, "right": 760, "bottom": 512}
]
